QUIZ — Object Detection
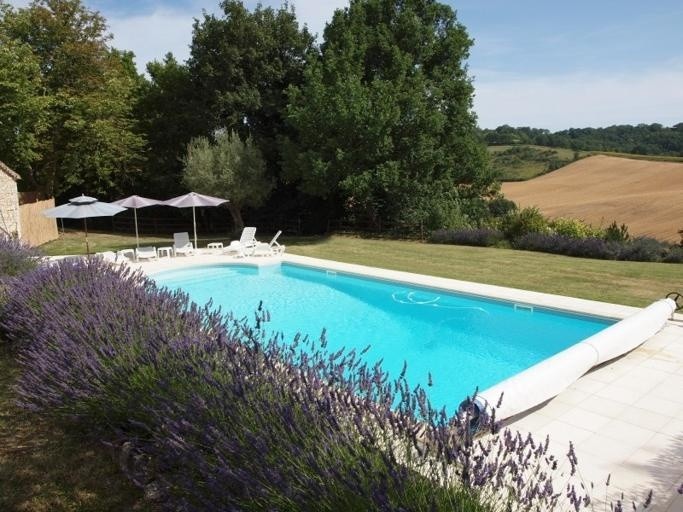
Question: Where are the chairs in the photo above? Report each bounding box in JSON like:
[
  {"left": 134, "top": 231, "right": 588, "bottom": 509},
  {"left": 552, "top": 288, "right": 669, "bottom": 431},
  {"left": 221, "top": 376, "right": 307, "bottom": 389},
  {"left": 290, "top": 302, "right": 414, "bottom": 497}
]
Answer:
[
  {"left": 136, "top": 246, "right": 157, "bottom": 263},
  {"left": 224, "top": 227, "right": 285, "bottom": 258},
  {"left": 172, "top": 232, "right": 194, "bottom": 257}
]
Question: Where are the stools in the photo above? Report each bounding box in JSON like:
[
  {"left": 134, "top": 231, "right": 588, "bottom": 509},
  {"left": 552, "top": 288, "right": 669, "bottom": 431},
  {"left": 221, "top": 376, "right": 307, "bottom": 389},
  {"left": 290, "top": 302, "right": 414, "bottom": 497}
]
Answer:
[
  {"left": 158, "top": 246, "right": 172, "bottom": 258},
  {"left": 207, "top": 242, "right": 222, "bottom": 253},
  {"left": 117, "top": 248, "right": 135, "bottom": 263},
  {"left": 95, "top": 252, "right": 117, "bottom": 264}
]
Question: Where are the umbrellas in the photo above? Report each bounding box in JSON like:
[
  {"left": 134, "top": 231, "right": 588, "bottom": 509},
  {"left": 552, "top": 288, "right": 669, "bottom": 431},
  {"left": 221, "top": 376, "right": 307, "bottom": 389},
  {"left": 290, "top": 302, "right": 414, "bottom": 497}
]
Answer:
[
  {"left": 107, "top": 194, "right": 169, "bottom": 247},
  {"left": 43, "top": 192, "right": 128, "bottom": 259},
  {"left": 161, "top": 190, "right": 231, "bottom": 250}
]
